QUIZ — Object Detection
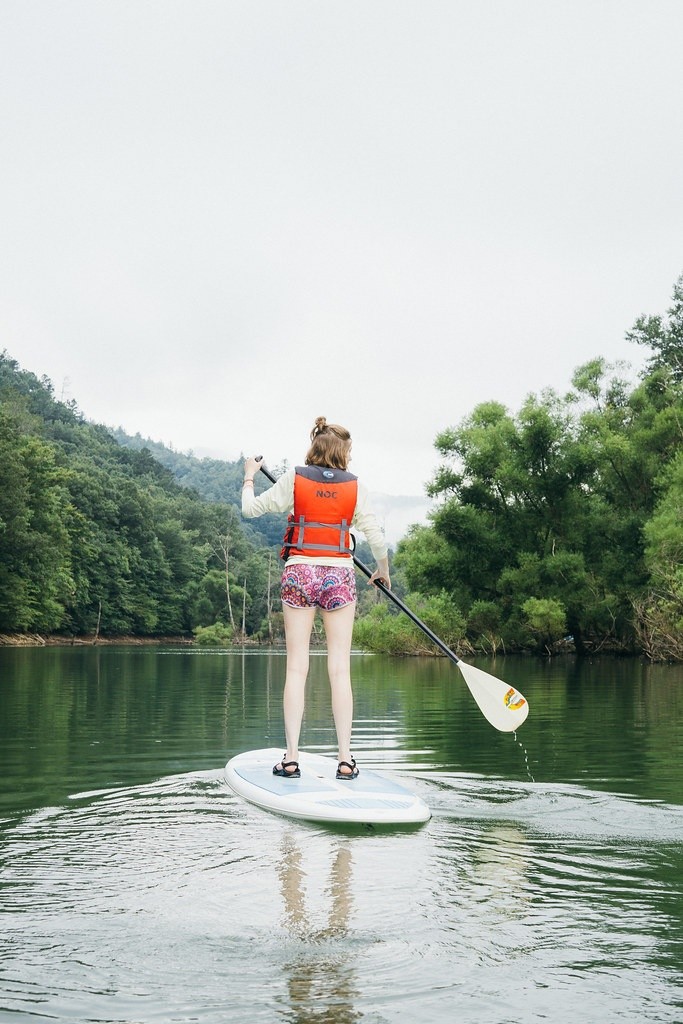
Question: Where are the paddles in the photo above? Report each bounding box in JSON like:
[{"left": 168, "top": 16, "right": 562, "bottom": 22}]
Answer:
[{"left": 254, "top": 453, "right": 530, "bottom": 735}]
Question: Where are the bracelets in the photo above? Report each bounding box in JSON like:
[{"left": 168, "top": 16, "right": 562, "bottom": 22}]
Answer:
[{"left": 243, "top": 479, "right": 254, "bottom": 484}]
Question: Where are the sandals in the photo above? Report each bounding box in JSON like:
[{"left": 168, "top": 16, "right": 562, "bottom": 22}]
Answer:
[
  {"left": 273, "top": 761, "right": 301, "bottom": 778},
  {"left": 336, "top": 760, "right": 359, "bottom": 780}
]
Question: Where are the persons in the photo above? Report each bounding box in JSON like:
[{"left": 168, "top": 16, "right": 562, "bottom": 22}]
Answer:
[{"left": 241, "top": 417, "right": 391, "bottom": 780}]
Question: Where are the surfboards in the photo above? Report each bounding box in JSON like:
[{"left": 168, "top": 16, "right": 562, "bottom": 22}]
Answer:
[{"left": 222, "top": 747, "right": 433, "bottom": 825}]
